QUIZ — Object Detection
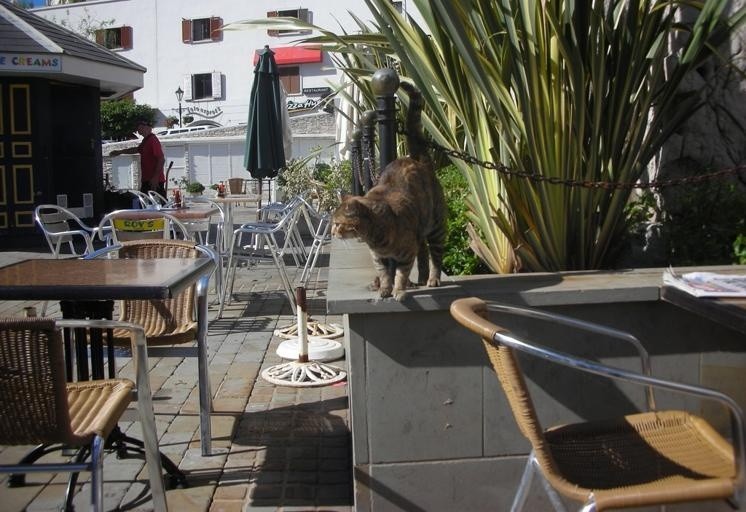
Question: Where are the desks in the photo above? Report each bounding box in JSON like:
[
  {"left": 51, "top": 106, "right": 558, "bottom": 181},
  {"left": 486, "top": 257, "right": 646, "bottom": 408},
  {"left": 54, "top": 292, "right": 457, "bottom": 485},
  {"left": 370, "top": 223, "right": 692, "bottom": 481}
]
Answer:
[
  {"left": 662, "top": 281, "right": 743, "bottom": 324},
  {"left": 1, "top": 257, "right": 215, "bottom": 488}
]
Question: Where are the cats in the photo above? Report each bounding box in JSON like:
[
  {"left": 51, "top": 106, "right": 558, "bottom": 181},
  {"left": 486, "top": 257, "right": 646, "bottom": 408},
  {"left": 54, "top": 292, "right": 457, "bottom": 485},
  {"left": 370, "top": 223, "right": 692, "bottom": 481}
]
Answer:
[{"left": 330, "top": 81, "right": 451, "bottom": 306}]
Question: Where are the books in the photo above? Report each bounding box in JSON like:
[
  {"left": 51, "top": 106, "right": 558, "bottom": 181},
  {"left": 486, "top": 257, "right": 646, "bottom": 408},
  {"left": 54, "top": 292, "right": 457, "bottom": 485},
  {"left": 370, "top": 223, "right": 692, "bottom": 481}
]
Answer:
[{"left": 663, "top": 264, "right": 746, "bottom": 298}]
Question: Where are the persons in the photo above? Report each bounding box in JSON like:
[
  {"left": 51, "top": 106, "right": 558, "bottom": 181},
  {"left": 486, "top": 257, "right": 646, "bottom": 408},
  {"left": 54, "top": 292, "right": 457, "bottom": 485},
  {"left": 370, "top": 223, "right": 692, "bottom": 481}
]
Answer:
[{"left": 109, "top": 118, "right": 167, "bottom": 208}]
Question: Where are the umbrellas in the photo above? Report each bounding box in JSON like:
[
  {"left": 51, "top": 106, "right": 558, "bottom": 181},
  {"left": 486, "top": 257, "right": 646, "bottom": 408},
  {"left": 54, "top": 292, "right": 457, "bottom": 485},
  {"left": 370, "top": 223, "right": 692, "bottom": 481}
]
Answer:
[{"left": 243, "top": 45, "right": 286, "bottom": 220}]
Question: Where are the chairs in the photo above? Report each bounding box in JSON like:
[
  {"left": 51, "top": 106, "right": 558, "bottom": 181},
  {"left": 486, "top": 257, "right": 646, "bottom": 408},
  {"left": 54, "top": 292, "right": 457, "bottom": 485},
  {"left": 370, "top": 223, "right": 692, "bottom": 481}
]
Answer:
[
  {"left": 70, "top": 239, "right": 215, "bottom": 457},
  {"left": 450, "top": 297, "right": 742, "bottom": 510},
  {"left": 33, "top": 190, "right": 312, "bottom": 320},
  {"left": 0, "top": 318, "right": 167, "bottom": 511}
]
